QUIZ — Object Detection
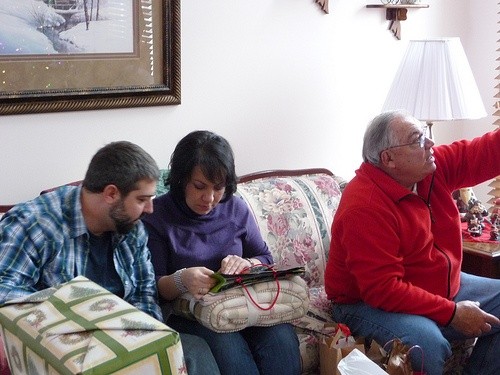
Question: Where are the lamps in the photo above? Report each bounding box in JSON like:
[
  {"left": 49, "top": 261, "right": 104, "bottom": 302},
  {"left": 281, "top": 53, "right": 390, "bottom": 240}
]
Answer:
[{"left": 385, "top": 36, "right": 488, "bottom": 143}]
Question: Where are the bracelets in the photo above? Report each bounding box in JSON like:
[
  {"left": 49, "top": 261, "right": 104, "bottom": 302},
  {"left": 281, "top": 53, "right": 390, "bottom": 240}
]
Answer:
[
  {"left": 171, "top": 267, "right": 188, "bottom": 294},
  {"left": 245, "top": 257, "right": 254, "bottom": 268}
]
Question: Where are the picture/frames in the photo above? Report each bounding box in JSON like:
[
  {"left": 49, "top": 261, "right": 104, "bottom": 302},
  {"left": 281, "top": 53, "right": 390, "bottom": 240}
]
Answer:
[{"left": 0, "top": 0, "right": 183, "bottom": 115}]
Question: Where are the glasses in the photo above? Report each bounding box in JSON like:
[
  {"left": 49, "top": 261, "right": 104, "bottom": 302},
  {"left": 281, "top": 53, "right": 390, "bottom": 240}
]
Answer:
[{"left": 379, "top": 128, "right": 429, "bottom": 159}]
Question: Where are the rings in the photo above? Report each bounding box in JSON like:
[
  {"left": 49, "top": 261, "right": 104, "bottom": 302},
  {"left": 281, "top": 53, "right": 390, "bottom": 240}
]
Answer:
[{"left": 200, "top": 289, "right": 203, "bottom": 294}]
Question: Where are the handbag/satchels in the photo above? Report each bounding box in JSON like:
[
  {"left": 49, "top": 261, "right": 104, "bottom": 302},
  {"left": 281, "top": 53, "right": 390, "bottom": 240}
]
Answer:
[
  {"left": 381, "top": 337, "right": 424, "bottom": 375},
  {"left": 209, "top": 262, "right": 306, "bottom": 310},
  {"left": 318, "top": 323, "right": 366, "bottom": 375}
]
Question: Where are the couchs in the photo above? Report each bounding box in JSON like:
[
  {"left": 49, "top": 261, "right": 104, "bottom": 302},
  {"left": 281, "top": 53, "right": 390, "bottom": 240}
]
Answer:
[{"left": 0, "top": 168, "right": 477, "bottom": 375}]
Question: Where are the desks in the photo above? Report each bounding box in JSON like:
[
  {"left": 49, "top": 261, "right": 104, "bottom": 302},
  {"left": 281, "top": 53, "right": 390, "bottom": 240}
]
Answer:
[{"left": 460, "top": 216, "right": 500, "bottom": 279}]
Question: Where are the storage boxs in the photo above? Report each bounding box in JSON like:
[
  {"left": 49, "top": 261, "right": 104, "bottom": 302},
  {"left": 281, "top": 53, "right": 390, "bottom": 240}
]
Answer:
[{"left": 0, "top": 275, "right": 189, "bottom": 375}]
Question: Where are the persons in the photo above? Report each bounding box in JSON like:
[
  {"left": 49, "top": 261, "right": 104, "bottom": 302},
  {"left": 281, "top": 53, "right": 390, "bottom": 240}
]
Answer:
[
  {"left": 142, "top": 130, "right": 303, "bottom": 375},
  {"left": 325, "top": 110, "right": 500, "bottom": 375},
  {"left": 0, "top": 140, "right": 221, "bottom": 375}
]
